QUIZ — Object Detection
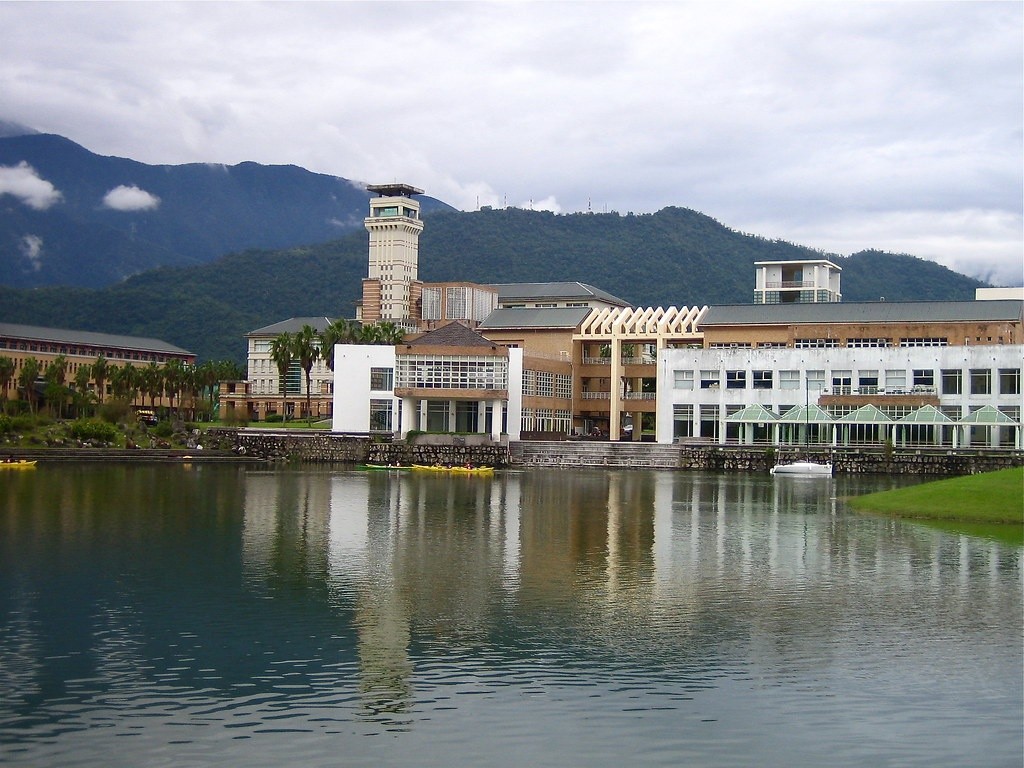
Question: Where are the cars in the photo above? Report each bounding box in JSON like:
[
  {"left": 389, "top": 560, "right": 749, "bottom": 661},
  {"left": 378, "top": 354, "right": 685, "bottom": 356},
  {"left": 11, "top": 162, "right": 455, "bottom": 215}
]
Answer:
[
  {"left": 138, "top": 415, "right": 157, "bottom": 427},
  {"left": 624, "top": 424, "right": 633, "bottom": 433},
  {"left": 363, "top": 459, "right": 496, "bottom": 473}
]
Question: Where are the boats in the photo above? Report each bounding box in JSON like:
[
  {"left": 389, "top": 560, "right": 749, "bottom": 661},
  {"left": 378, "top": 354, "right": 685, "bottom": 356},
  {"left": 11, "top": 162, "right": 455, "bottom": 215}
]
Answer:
[
  {"left": 770, "top": 459, "right": 834, "bottom": 476},
  {"left": 0, "top": 454, "right": 37, "bottom": 466}
]
